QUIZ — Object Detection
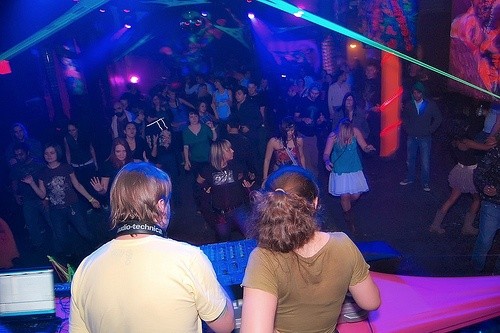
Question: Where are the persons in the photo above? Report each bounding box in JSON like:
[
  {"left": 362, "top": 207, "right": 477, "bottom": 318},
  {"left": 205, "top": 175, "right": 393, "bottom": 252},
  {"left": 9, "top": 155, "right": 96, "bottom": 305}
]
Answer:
[
  {"left": 69, "top": 161, "right": 235, "bottom": 333},
  {"left": 238, "top": 167, "right": 380, "bottom": 333},
  {"left": 400, "top": 56, "right": 500, "bottom": 279},
  {"left": 0, "top": 10, "right": 381, "bottom": 269}
]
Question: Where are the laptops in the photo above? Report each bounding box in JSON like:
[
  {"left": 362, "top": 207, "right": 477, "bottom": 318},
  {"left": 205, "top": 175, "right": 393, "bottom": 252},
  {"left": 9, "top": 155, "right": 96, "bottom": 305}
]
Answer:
[{"left": 0, "top": 266, "right": 58, "bottom": 333}]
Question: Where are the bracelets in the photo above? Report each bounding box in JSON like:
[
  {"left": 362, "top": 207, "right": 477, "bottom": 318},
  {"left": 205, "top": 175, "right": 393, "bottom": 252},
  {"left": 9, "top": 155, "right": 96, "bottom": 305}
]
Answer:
[
  {"left": 89, "top": 197, "right": 93, "bottom": 202},
  {"left": 323, "top": 160, "right": 330, "bottom": 164},
  {"left": 185, "top": 159, "right": 188, "bottom": 160},
  {"left": 211, "top": 127, "right": 215, "bottom": 130}
]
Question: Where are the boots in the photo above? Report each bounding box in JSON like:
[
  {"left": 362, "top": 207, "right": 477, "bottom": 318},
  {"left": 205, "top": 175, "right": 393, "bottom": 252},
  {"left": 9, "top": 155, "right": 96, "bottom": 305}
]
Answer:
[
  {"left": 428, "top": 209, "right": 445, "bottom": 235},
  {"left": 460, "top": 212, "right": 479, "bottom": 235}
]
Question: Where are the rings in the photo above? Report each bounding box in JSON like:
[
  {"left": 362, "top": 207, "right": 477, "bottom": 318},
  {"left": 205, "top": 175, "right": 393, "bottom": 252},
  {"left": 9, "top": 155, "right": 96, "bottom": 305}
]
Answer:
[
  {"left": 488, "top": 189, "right": 489, "bottom": 192},
  {"left": 488, "top": 186, "right": 490, "bottom": 189}
]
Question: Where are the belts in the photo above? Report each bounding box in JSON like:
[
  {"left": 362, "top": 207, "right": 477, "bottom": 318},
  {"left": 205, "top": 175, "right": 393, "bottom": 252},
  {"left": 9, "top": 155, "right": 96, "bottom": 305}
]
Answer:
[{"left": 213, "top": 201, "right": 244, "bottom": 214}]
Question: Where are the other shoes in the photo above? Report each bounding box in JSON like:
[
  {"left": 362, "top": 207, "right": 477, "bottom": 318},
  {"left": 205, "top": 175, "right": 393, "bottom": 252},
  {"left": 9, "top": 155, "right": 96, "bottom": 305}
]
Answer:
[
  {"left": 423, "top": 184, "right": 431, "bottom": 191},
  {"left": 400, "top": 178, "right": 413, "bottom": 185}
]
